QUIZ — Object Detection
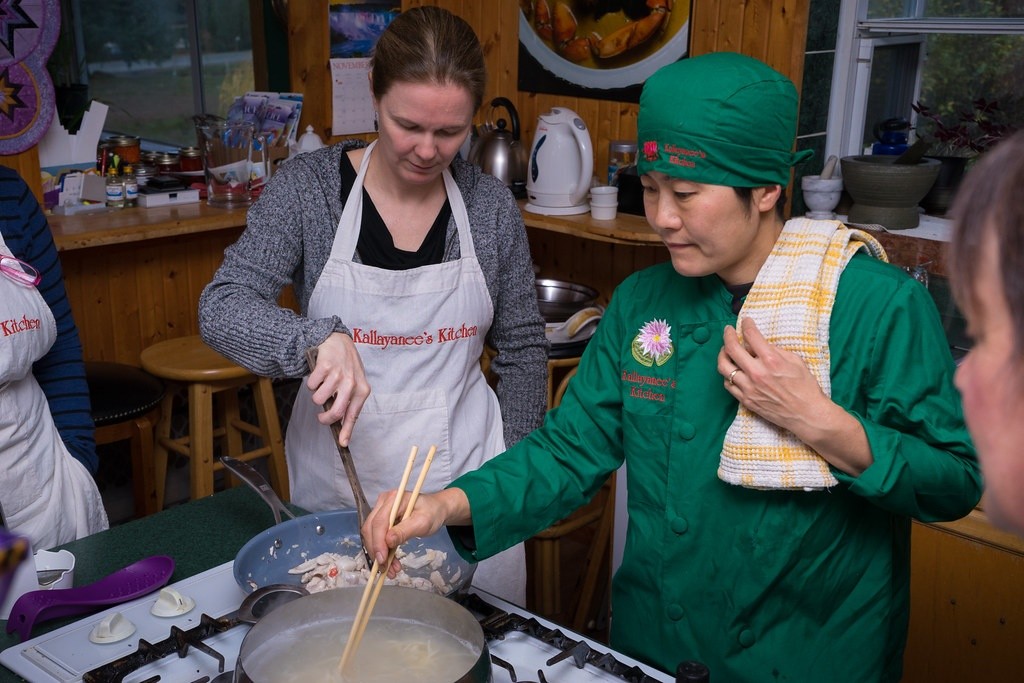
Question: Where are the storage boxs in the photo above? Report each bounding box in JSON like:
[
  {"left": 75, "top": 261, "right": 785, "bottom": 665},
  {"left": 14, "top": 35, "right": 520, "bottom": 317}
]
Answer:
[{"left": 206, "top": 137, "right": 289, "bottom": 178}]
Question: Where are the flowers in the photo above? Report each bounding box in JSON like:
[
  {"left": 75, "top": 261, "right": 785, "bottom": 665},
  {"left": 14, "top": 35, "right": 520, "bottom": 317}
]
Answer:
[{"left": 904, "top": 101, "right": 1001, "bottom": 160}]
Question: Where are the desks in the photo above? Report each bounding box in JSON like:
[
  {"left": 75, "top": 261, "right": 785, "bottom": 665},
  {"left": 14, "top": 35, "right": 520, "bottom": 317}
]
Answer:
[{"left": 0, "top": 486, "right": 312, "bottom": 683}]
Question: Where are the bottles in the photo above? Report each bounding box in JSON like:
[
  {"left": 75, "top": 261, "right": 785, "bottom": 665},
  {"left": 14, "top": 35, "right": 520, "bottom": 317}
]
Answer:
[{"left": 106, "top": 166, "right": 137, "bottom": 208}]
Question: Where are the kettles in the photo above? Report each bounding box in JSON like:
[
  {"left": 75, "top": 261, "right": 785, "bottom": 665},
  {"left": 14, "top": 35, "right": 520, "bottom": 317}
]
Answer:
[
  {"left": 466, "top": 96, "right": 528, "bottom": 199},
  {"left": 523, "top": 105, "right": 591, "bottom": 215}
]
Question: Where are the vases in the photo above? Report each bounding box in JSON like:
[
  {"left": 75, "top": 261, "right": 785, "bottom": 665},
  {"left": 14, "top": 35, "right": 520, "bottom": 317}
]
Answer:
[{"left": 907, "top": 147, "right": 978, "bottom": 215}]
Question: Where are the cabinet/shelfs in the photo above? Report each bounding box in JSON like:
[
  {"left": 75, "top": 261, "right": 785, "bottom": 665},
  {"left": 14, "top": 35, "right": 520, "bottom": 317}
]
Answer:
[{"left": 481, "top": 348, "right": 616, "bottom": 643}]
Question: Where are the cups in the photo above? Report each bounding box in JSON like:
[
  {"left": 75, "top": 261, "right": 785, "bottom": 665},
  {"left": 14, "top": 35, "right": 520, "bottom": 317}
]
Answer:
[
  {"left": 291, "top": 126, "right": 329, "bottom": 160},
  {"left": 196, "top": 119, "right": 269, "bottom": 209},
  {"left": 608, "top": 143, "right": 640, "bottom": 186},
  {"left": 107, "top": 134, "right": 203, "bottom": 187},
  {"left": 590, "top": 187, "right": 618, "bottom": 220}
]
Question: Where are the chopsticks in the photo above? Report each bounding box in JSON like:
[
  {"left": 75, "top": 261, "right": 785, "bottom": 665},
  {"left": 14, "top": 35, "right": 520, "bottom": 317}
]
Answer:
[{"left": 337, "top": 445, "right": 437, "bottom": 673}]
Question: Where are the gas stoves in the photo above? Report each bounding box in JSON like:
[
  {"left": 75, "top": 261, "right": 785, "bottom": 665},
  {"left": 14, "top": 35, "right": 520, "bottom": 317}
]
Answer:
[{"left": 0, "top": 559, "right": 712, "bottom": 683}]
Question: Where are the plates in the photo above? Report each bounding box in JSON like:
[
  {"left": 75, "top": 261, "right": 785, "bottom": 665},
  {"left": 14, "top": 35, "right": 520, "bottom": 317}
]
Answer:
[{"left": 517, "top": 8, "right": 688, "bottom": 89}]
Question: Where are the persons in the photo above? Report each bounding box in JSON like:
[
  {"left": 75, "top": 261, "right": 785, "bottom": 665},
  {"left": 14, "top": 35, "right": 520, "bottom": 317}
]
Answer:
[
  {"left": 1, "top": 161, "right": 119, "bottom": 598},
  {"left": 943, "top": 131, "right": 1024, "bottom": 531},
  {"left": 357, "top": 50, "right": 984, "bottom": 683},
  {"left": 197, "top": 8, "right": 551, "bottom": 610}
]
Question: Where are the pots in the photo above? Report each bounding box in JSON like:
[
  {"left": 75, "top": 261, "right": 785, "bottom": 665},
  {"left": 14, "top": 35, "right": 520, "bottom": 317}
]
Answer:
[
  {"left": 232, "top": 586, "right": 494, "bottom": 683},
  {"left": 220, "top": 455, "right": 479, "bottom": 607}
]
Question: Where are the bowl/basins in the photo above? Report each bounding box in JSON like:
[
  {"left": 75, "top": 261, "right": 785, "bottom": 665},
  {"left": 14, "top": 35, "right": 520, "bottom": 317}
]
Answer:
[{"left": 533, "top": 277, "right": 600, "bottom": 323}]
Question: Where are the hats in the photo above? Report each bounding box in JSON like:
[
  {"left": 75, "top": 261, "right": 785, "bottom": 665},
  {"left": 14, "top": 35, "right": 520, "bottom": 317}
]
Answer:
[{"left": 636, "top": 50, "right": 814, "bottom": 190}]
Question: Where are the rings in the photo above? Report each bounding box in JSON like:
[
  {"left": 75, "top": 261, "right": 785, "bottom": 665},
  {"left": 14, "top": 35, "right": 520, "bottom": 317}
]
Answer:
[{"left": 729, "top": 369, "right": 741, "bottom": 386}]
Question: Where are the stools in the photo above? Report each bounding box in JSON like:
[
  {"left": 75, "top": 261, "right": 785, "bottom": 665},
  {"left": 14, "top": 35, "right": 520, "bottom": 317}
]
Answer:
[
  {"left": 89, "top": 360, "right": 177, "bottom": 517},
  {"left": 142, "top": 331, "right": 288, "bottom": 524}
]
